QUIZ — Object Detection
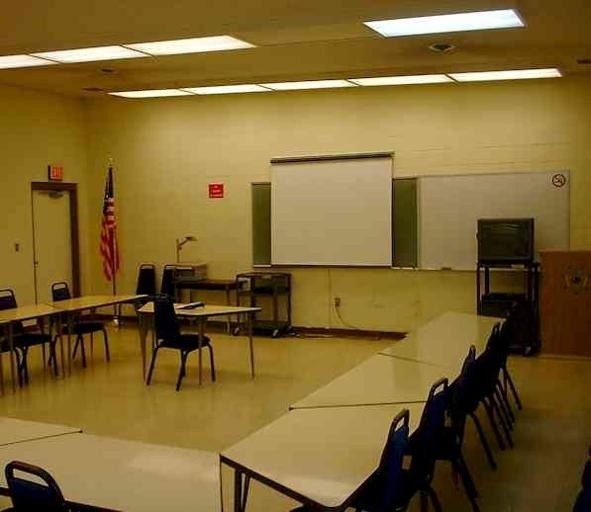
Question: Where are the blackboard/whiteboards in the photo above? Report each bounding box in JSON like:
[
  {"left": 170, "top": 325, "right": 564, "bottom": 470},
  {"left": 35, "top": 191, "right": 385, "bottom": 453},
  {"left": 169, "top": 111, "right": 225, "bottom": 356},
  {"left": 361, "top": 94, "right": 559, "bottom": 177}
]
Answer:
[
  {"left": 417, "top": 169, "right": 570, "bottom": 273},
  {"left": 252, "top": 176, "right": 417, "bottom": 270}
]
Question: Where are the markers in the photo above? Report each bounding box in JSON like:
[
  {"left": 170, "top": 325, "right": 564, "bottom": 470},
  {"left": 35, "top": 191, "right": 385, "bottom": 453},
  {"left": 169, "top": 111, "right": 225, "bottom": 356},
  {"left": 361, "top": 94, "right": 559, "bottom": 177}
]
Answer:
[{"left": 441, "top": 267, "right": 451, "bottom": 270}]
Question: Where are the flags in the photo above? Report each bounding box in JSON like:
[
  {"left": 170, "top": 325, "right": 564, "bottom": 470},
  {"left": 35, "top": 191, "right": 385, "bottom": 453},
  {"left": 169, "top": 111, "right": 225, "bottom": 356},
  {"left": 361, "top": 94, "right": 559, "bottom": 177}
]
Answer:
[{"left": 100, "top": 158, "right": 120, "bottom": 282}]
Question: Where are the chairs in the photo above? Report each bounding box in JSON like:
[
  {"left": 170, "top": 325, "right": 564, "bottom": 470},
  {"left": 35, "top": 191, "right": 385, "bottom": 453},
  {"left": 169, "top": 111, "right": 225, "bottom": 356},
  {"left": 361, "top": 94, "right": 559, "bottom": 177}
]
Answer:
[
  {"left": 46, "top": 280, "right": 113, "bottom": 370},
  {"left": 0, "top": 286, "right": 66, "bottom": 388},
  {"left": 144, "top": 296, "right": 217, "bottom": 392},
  {"left": 4, "top": 458, "right": 71, "bottom": 511},
  {"left": 345, "top": 309, "right": 523, "bottom": 512},
  {"left": 160, "top": 261, "right": 180, "bottom": 301},
  {"left": 136, "top": 262, "right": 155, "bottom": 303}
]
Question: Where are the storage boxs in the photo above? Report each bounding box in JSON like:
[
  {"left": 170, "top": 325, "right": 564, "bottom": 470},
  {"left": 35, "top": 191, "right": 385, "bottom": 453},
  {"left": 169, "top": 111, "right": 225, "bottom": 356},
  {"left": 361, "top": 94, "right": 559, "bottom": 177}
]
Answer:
[{"left": 166, "top": 261, "right": 208, "bottom": 282}]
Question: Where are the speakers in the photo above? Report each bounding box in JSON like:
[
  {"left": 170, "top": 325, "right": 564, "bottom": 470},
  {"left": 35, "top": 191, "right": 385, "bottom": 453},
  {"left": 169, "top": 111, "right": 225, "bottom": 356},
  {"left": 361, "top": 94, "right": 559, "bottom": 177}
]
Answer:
[{"left": 483, "top": 293, "right": 530, "bottom": 349}]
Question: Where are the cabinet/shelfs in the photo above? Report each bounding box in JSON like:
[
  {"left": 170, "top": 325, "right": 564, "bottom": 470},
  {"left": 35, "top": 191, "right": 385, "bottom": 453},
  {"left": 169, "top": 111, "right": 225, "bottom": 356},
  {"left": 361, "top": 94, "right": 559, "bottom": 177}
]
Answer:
[
  {"left": 471, "top": 262, "right": 541, "bottom": 357},
  {"left": 232, "top": 271, "right": 294, "bottom": 339}
]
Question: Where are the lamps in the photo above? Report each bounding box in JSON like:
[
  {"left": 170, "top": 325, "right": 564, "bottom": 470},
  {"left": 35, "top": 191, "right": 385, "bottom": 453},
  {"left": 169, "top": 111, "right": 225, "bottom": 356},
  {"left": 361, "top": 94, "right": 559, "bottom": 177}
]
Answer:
[{"left": 174, "top": 231, "right": 196, "bottom": 262}]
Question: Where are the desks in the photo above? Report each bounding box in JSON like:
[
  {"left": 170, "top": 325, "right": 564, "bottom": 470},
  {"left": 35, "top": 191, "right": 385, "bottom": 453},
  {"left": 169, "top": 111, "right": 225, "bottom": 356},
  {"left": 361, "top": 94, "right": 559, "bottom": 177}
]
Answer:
[
  {"left": 218, "top": 403, "right": 426, "bottom": 512},
  {"left": 378, "top": 311, "right": 506, "bottom": 371},
  {"left": 179, "top": 274, "right": 248, "bottom": 336},
  {"left": 0, "top": 433, "right": 302, "bottom": 511},
  {"left": 289, "top": 355, "right": 461, "bottom": 409},
  {"left": 138, "top": 299, "right": 262, "bottom": 380},
  {"left": 1, "top": 415, "right": 83, "bottom": 446},
  {"left": 46, "top": 292, "right": 149, "bottom": 374}
]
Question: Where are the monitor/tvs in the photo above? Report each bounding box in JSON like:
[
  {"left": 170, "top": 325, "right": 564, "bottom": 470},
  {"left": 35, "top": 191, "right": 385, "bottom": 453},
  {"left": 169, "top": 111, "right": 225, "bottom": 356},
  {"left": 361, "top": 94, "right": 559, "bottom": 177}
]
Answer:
[{"left": 477, "top": 218, "right": 535, "bottom": 263}]
